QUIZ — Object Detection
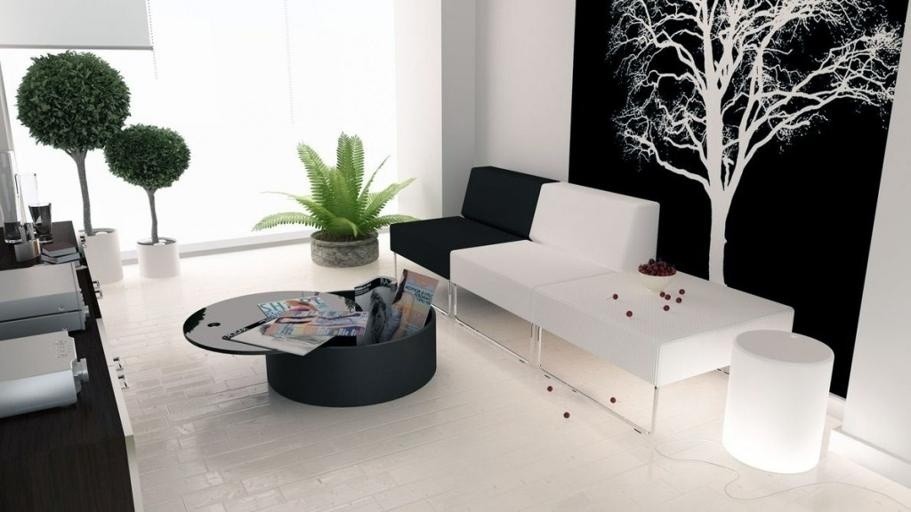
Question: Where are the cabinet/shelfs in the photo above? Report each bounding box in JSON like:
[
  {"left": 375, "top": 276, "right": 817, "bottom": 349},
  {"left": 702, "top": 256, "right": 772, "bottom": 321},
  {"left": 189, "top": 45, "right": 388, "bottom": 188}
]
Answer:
[{"left": 0, "top": 221, "right": 145, "bottom": 512}]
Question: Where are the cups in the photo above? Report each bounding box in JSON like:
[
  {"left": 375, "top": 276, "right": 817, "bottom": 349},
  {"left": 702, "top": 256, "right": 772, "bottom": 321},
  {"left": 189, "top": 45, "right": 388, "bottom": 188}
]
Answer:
[
  {"left": 1, "top": 149, "right": 29, "bottom": 244},
  {"left": 28, "top": 202, "right": 56, "bottom": 243}
]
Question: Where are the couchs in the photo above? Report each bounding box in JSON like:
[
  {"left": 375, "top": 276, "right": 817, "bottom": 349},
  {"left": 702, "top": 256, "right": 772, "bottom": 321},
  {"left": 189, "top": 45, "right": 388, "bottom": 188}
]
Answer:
[{"left": 390, "top": 165, "right": 795, "bottom": 436}]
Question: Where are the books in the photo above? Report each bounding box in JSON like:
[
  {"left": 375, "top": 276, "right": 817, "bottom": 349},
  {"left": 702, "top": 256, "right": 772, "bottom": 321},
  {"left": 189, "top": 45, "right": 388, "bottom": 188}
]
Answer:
[
  {"left": 40, "top": 242, "right": 81, "bottom": 268},
  {"left": 222, "top": 268, "right": 439, "bottom": 358}
]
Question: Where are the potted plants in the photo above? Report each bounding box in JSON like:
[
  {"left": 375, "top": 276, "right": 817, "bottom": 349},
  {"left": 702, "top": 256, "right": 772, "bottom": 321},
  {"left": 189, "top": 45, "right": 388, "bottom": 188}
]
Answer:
[
  {"left": 251, "top": 132, "right": 421, "bottom": 268},
  {"left": 103, "top": 123, "right": 191, "bottom": 278},
  {"left": 15, "top": 48, "right": 133, "bottom": 284}
]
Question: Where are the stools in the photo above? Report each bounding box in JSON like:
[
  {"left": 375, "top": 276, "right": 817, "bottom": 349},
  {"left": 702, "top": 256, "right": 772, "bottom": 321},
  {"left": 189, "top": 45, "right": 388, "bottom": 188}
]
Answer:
[{"left": 721, "top": 330, "right": 835, "bottom": 474}]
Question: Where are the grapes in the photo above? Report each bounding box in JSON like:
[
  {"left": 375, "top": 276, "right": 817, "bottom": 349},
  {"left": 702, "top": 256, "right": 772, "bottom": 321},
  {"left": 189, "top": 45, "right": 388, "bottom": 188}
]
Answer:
[
  {"left": 564, "top": 411, "right": 569, "bottom": 418},
  {"left": 547, "top": 386, "right": 553, "bottom": 391},
  {"left": 612, "top": 257, "right": 686, "bottom": 318},
  {"left": 611, "top": 397, "right": 616, "bottom": 403}
]
